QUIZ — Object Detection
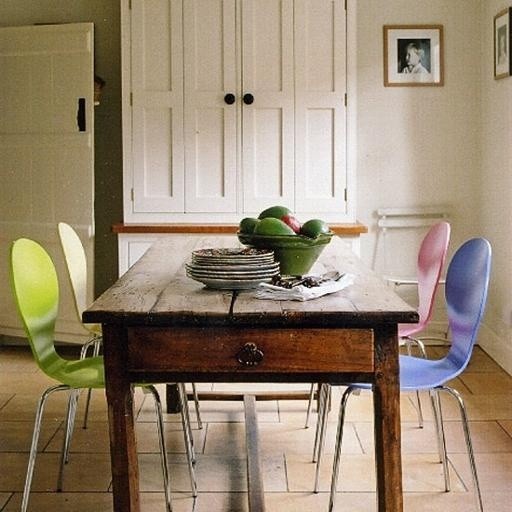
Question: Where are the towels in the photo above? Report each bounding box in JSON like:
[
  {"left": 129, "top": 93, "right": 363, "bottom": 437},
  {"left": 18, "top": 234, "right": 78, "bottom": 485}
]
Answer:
[{"left": 255, "top": 273, "right": 355, "bottom": 302}]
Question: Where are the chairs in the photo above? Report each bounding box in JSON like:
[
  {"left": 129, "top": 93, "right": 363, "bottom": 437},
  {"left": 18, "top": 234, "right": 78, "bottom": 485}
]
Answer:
[
  {"left": 58, "top": 220, "right": 202, "bottom": 465},
  {"left": 11, "top": 237, "right": 197, "bottom": 512},
  {"left": 314, "top": 238, "right": 492, "bottom": 512},
  {"left": 304, "top": 220, "right": 451, "bottom": 463}
]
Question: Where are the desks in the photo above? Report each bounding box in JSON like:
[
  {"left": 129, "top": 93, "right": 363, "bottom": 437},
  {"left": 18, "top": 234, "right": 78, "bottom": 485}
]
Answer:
[{"left": 82, "top": 235, "right": 420, "bottom": 512}]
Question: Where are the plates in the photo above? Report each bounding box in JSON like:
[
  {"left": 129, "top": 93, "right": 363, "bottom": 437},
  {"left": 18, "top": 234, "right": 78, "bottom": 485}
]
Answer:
[{"left": 185, "top": 247, "right": 281, "bottom": 290}]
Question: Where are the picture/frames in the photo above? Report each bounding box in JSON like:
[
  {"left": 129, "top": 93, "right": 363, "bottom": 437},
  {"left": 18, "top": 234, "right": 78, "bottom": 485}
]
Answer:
[
  {"left": 383, "top": 25, "right": 444, "bottom": 87},
  {"left": 494, "top": 7, "right": 512, "bottom": 80}
]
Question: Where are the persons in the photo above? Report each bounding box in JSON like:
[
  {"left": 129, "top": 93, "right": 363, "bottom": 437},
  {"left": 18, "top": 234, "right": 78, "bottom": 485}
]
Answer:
[
  {"left": 498, "top": 32, "right": 508, "bottom": 63},
  {"left": 400, "top": 41, "right": 431, "bottom": 73}
]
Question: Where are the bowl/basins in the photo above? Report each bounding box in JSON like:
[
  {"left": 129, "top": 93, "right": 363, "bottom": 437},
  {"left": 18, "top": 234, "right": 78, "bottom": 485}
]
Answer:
[{"left": 236, "top": 233, "right": 337, "bottom": 278}]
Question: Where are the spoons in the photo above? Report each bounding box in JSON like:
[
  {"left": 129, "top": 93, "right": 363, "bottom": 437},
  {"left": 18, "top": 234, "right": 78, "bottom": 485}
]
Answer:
[{"left": 305, "top": 271, "right": 346, "bottom": 288}]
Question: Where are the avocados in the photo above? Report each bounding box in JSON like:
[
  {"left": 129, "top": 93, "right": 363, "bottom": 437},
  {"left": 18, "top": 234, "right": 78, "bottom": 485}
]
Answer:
[{"left": 239, "top": 205, "right": 329, "bottom": 239}]
